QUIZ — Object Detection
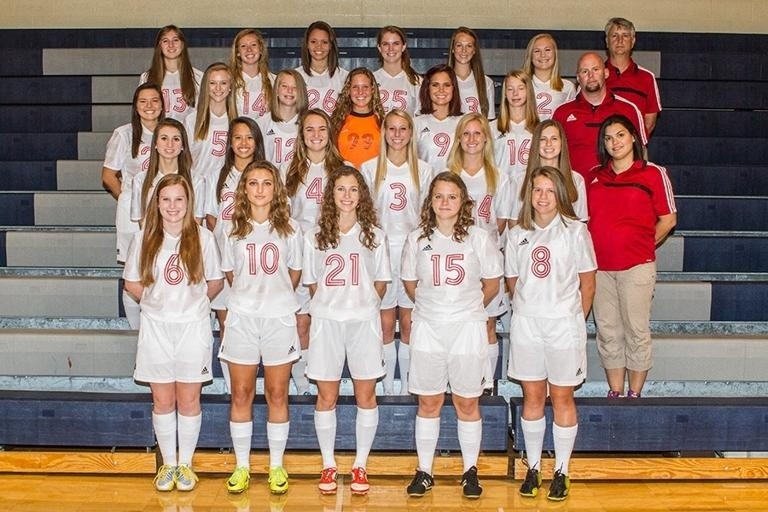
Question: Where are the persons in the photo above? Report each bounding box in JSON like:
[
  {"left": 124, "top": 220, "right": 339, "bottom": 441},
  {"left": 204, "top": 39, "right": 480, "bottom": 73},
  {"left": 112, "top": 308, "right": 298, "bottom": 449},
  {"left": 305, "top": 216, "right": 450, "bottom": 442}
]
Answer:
[
  {"left": 217, "top": 160, "right": 302, "bottom": 492},
  {"left": 122, "top": 174, "right": 224, "bottom": 490},
  {"left": 553, "top": 17, "right": 676, "bottom": 397},
  {"left": 102, "top": 21, "right": 588, "bottom": 396},
  {"left": 505, "top": 166, "right": 599, "bottom": 500},
  {"left": 400, "top": 171, "right": 504, "bottom": 498},
  {"left": 302, "top": 166, "right": 392, "bottom": 495}
]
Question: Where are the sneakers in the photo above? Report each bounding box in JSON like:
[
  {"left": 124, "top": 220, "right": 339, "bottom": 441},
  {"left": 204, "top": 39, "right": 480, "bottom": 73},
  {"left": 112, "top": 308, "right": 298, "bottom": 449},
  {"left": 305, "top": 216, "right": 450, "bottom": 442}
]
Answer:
[
  {"left": 459, "top": 466, "right": 483, "bottom": 498},
  {"left": 406, "top": 466, "right": 435, "bottom": 497},
  {"left": 546, "top": 462, "right": 570, "bottom": 501},
  {"left": 520, "top": 458, "right": 542, "bottom": 497},
  {"left": 267, "top": 466, "right": 289, "bottom": 495},
  {"left": 170, "top": 464, "right": 198, "bottom": 492},
  {"left": 151, "top": 466, "right": 175, "bottom": 492},
  {"left": 226, "top": 466, "right": 249, "bottom": 494},
  {"left": 318, "top": 467, "right": 338, "bottom": 496},
  {"left": 350, "top": 467, "right": 369, "bottom": 496}
]
